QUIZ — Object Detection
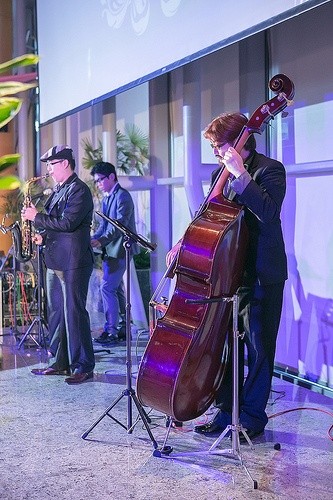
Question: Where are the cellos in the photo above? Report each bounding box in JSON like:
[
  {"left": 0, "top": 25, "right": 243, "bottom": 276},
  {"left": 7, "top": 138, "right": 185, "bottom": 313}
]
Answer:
[{"left": 134, "top": 71, "right": 297, "bottom": 458}]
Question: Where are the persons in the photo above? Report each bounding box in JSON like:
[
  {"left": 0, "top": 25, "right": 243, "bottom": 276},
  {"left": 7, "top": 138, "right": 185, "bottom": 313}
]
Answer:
[
  {"left": 165, "top": 112, "right": 289, "bottom": 442},
  {"left": 21, "top": 144, "right": 94, "bottom": 384},
  {"left": 90, "top": 161, "right": 141, "bottom": 347}
]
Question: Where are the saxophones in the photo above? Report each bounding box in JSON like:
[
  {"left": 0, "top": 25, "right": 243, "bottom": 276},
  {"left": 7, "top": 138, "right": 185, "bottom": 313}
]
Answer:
[{"left": 0, "top": 172, "right": 50, "bottom": 264}]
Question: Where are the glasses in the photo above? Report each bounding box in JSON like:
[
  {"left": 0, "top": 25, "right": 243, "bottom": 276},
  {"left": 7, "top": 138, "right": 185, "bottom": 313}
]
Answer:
[
  {"left": 94, "top": 176, "right": 107, "bottom": 184},
  {"left": 209, "top": 141, "right": 227, "bottom": 149},
  {"left": 47, "top": 159, "right": 64, "bottom": 167}
]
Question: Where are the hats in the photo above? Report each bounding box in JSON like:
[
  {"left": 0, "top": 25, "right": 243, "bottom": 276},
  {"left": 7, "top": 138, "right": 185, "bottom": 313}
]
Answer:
[{"left": 40, "top": 144, "right": 74, "bottom": 162}]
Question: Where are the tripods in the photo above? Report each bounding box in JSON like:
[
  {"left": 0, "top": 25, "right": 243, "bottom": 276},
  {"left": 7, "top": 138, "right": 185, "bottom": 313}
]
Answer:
[
  {"left": 201, "top": 285, "right": 281, "bottom": 491},
  {"left": 0, "top": 229, "right": 52, "bottom": 353},
  {"left": 81, "top": 242, "right": 161, "bottom": 458}
]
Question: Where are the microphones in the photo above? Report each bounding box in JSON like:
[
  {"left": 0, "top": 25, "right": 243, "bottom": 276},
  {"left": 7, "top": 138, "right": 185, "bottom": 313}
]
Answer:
[{"left": 30, "top": 188, "right": 52, "bottom": 200}]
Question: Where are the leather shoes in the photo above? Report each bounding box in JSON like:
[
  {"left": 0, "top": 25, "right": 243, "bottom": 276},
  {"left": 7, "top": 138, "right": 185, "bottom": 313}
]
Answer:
[
  {"left": 65, "top": 368, "right": 94, "bottom": 383},
  {"left": 30, "top": 366, "right": 69, "bottom": 375},
  {"left": 91, "top": 332, "right": 117, "bottom": 344},
  {"left": 116, "top": 331, "right": 131, "bottom": 342},
  {"left": 229, "top": 427, "right": 265, "bottom": 442},
  {"left": 194, "top": 420, "right": 224, "bottom": 434}
]
